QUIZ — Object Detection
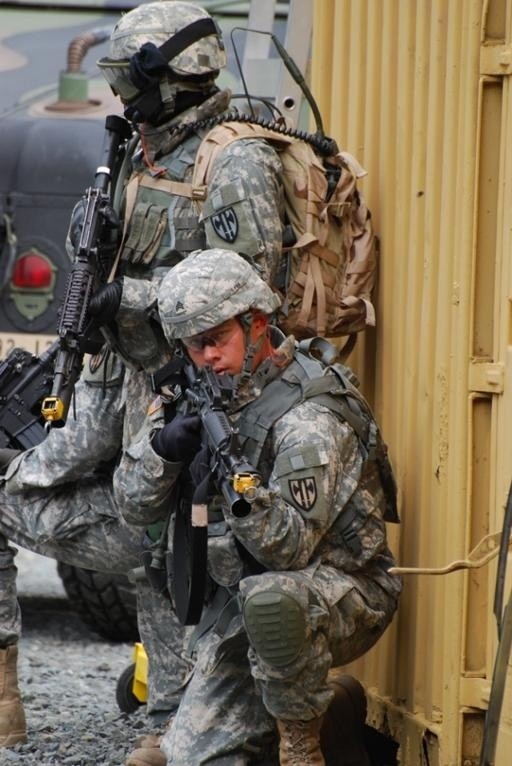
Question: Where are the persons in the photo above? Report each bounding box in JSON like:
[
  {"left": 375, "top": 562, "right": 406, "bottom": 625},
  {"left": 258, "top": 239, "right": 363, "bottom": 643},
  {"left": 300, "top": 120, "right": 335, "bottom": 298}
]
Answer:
[
  {"left": 0, "top": 0, "right": 286, "bottom": 766},
  {"left": 114, "top": 248, "right": 402, "bottom": 766}
]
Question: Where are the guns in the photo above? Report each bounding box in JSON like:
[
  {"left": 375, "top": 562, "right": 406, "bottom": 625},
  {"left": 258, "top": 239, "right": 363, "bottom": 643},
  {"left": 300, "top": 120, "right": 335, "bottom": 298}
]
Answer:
[
  {"left": 42, "top": 115, "right": 132, "bottom": 433},
  {"left": 152, "top": 352, "right": 262, "bottom": 518},
  {"left": 1, "top": 340, "right": 63, "bottom": 450}
]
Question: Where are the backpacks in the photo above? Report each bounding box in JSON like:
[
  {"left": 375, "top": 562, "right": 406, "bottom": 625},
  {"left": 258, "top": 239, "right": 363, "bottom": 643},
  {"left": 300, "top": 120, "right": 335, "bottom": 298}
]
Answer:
[{"left": 188, "top": 121, "right": 377, "bottom": 339}]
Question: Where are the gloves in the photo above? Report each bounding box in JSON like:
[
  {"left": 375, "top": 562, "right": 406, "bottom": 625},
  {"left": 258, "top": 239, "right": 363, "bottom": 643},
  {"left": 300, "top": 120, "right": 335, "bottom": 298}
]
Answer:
[
  {"left": 156, "top": 408, "right": 230, "bottom": 502},
  {"left": 67, "top": 197, "right": 125, "bottom": 342}
]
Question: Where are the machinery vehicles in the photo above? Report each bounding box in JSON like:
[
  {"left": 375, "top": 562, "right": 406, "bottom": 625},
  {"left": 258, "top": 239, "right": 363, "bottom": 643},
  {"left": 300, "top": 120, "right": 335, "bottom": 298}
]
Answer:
[{"left": 1, "top": 1, "right": 286, "bottom": 641}]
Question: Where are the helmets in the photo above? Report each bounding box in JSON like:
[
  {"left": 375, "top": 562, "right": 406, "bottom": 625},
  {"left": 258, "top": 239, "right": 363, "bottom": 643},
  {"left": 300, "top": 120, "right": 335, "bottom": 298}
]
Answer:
[
  {"left": 107, "top": 1, "right": 226, "bottom": 74},
  {"left": 158, "top": 249, "right": 285, "bottom": 345}
]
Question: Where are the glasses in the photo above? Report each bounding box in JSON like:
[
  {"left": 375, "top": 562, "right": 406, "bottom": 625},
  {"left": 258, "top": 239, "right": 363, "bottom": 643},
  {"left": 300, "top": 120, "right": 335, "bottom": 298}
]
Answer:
[
  {"left": 96, "top": 58, "right": 145, "bottom": 99},
  {"left": 180, "top": 327, "right": 239, "bottom": 351}
]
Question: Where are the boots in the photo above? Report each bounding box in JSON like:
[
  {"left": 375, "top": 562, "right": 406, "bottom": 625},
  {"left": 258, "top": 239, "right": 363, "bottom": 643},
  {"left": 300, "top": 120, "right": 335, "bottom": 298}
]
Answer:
[
  {"left": 0, "top": 644, "right": 27, "bottom": 745},
  {"left": 271, "top": 674, "right": 369, "bottom": 766},
  {"left": 125, "top": 732, "right": 171, "bottom": 766}
]
